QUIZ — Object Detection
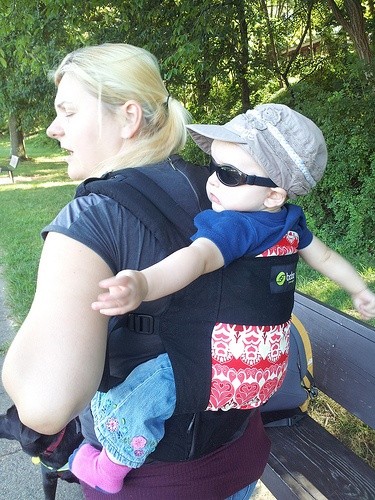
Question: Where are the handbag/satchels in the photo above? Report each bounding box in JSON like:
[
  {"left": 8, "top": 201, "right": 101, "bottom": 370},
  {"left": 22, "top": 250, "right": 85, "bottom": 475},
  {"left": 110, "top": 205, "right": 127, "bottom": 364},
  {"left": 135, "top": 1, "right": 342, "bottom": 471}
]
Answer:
[{"left": 261, "top": 313, "right": 318, "bottom": 426}]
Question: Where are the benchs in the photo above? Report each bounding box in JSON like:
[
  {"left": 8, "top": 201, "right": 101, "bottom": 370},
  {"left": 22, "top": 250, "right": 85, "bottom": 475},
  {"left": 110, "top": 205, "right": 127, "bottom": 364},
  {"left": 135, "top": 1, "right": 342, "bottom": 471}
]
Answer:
[{"left": 260, "top": 289, "right": 375, "bottom": 500}]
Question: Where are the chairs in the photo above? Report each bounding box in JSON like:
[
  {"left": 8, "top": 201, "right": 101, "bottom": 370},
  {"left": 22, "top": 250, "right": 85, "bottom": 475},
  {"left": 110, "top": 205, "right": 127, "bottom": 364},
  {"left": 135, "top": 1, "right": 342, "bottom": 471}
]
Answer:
[{"left": 0, "top": 155, "right": 19, "bottom": 183}]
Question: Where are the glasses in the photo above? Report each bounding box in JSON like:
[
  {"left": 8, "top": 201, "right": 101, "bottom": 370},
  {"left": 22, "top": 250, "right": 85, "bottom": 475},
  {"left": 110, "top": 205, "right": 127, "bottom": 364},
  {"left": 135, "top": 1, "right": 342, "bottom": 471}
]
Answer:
[{"left": 209, "top": 155, "right": 280, "bottom": 188}]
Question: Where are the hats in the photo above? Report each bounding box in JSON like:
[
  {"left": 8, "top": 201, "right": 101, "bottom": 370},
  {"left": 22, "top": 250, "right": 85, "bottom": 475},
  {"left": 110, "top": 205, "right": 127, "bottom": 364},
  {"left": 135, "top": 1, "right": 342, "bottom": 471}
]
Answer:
[{"left": 185, "top": 103, "right": 328, "bottom": 200}]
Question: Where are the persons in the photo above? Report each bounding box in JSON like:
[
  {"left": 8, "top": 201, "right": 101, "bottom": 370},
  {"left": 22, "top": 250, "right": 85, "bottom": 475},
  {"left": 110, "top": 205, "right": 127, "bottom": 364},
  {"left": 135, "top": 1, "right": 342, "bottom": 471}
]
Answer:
[
  {"left": 69, "top": 105, "right": 374, "bottom": 495},
  {"left": 1, "top": 44, "right": 267, "bottom": 500}
]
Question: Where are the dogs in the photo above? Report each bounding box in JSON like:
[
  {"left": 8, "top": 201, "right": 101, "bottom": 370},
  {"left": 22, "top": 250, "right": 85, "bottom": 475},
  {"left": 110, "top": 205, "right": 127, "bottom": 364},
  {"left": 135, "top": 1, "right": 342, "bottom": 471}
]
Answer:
[{"left": 0, "top": 403, "right": 85, "bottom": 500}]
